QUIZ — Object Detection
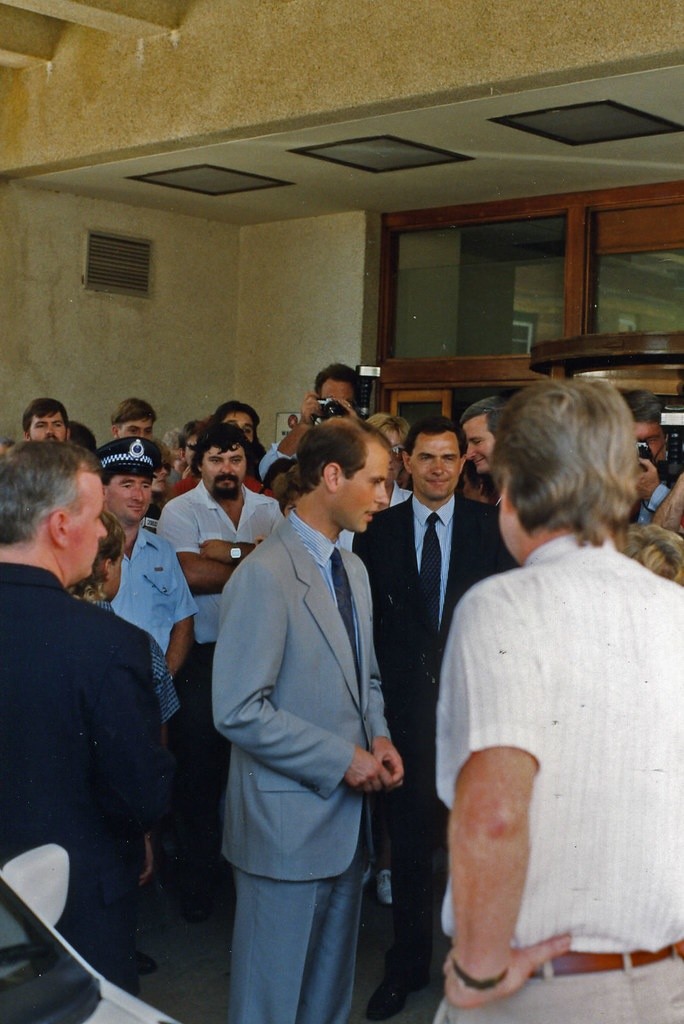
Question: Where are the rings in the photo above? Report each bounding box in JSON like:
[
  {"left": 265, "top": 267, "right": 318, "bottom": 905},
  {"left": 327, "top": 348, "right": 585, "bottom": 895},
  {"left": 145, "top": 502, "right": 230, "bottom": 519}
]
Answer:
[{"left": 348, "top": 403, "right": 351, "bottom": 407}]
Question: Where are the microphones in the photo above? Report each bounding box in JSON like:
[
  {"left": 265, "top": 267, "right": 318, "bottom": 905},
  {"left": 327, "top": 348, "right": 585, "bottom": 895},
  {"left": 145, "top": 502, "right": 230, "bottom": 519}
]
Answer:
[{"left": 141, "top": 503, "right": 161, "bottom": 533}]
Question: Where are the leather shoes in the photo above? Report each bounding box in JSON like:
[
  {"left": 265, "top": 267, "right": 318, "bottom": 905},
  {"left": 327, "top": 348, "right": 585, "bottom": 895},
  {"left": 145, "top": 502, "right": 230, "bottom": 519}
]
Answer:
[{"left": 366, "top": 966, "right": 431, "bottom": 1019}]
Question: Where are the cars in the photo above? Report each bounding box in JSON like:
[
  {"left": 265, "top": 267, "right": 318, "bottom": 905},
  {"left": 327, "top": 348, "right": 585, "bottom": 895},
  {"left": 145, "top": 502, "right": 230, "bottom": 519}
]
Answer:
[{"left": 1, "top": 839, "right": 183, "bottom": 1024}]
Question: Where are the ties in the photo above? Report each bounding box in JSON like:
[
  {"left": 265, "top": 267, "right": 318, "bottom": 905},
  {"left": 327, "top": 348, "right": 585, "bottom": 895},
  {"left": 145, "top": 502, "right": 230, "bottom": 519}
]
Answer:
[
  {"left": 418, "top": 511, "right": 441, "bottom": 647},
  {"left": 330, "top": 547, "right": 361, "bottom": 708}
]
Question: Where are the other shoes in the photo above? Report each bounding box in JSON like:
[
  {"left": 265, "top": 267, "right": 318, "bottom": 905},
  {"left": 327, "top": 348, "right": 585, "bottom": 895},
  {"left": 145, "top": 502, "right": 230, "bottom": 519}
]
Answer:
[
  {"left": 167, "top": 855, "right": 211, "bottom": 922},
  {"left": 135, "top": 950, "right": 157, "bottom": 975}
]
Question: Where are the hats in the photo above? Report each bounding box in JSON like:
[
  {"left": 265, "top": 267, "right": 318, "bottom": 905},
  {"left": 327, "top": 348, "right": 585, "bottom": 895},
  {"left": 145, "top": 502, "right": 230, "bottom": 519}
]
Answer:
[{"left": 96, "top": 436, "right": 161, "bottom": 480}]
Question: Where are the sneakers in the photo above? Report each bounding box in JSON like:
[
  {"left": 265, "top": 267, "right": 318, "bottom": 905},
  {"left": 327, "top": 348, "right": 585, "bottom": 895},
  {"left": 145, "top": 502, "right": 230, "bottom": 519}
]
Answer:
[
  {"left": 361, "top": 862, "right": 371, "bottom": 885},
  {"left": 375, "top": 869, "right": 394, "bottom": 905}
]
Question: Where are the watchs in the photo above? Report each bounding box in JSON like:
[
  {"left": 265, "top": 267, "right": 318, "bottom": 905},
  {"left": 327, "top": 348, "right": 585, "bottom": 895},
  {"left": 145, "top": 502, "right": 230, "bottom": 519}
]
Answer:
[{"left": 230, "top": 541, "right": 242, "bottom": 565}]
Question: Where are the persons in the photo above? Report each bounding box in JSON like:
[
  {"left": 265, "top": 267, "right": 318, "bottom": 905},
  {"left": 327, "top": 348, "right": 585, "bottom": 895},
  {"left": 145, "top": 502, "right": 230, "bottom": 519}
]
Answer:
[
  {"left": 212, "top": 414, "right": 405, "bottom": 1024},
  {"left": 335, "top": 412, "right": 413, "bottom": 552},
  {"left": 352, "top": 416, "right": 520, "bottom": 1024},
  {"left": 258, "top": 362, "right": 364, "bottom": 483},
  {"left": 0, "top": 438, "right": 170, "bottom": 996},
  {"left": 0, "top": 398, "right": 200, "bottom": 994},
  {"left": 459, "top": 396, "right": 505, "bottom": 505},
  {"left": 172, "top": 400, "right": 275, "bottom": 500},
  {"left": 615, "top": 524, "right": 684, "bottom": 588},
  {"left": 623, "top": 388, "right": 684, "bottom": 534},
  {"left": 434, "top": 380, "right": 684, "bottom": 1024},
  {"left": 155, "top": 424, "right": 284, "bottom": 924},
  {"left": 271, "top": 464, "right": 305, "bottom": 516}
]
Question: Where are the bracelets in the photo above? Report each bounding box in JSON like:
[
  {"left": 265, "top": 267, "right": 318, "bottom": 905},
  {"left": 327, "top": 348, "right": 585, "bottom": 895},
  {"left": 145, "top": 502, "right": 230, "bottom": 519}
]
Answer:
[{"left": 452, "top": 954, "right": 508, "bottom": 992}]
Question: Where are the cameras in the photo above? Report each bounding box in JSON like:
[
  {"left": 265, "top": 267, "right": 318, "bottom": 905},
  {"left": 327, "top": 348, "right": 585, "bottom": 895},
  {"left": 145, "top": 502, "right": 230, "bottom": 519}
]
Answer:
[
  {"left": 638, "top": 440, "right": 652, "bottom": 461},
  {"left": 313, "top": 397, "right": 346, "bottom": 419}
]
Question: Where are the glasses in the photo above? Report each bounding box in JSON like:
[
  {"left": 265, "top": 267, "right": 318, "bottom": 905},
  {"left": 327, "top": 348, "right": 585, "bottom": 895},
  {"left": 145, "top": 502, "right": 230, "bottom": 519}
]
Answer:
[
  {"left": 186, "top": 442, "right": 198, "bottom": 452},
  {"left": 392, "top": 444, "right": 406, "bottom": 462}
]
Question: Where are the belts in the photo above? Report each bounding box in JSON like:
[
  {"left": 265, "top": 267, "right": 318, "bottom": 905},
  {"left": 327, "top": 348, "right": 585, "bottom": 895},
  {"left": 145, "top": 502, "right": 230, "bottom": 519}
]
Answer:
[{"left": 527, "top": 942, "right": 684, "bottom": 978}]
